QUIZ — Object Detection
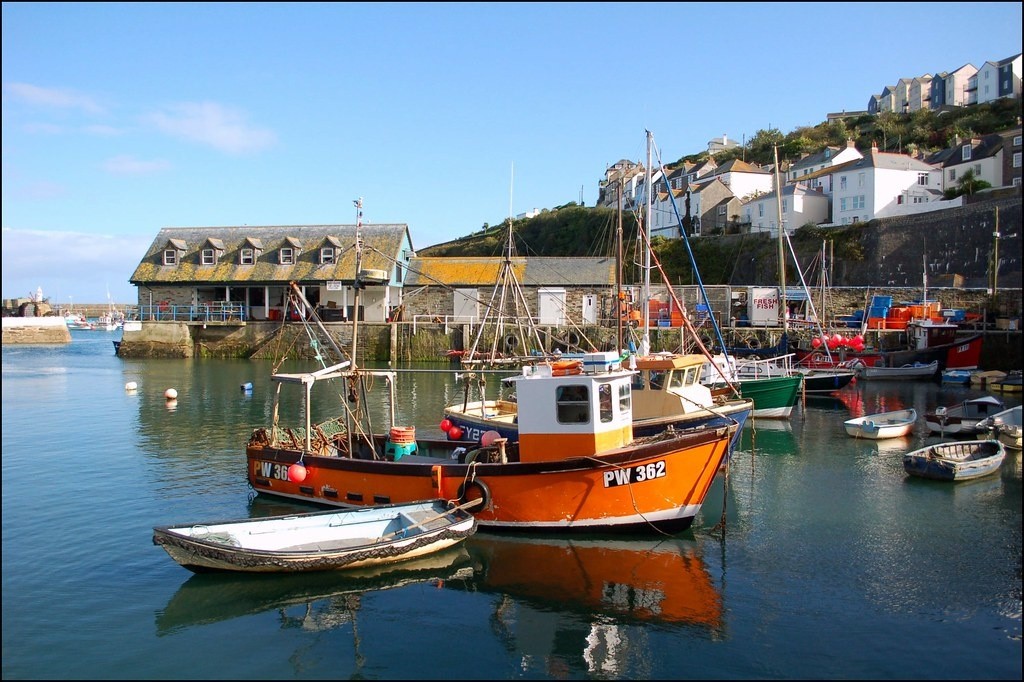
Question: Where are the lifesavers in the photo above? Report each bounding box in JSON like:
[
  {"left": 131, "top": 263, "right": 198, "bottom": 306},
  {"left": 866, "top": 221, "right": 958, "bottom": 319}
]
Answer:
[
  {"left": 457, "top": 478, "right": 490, "bottom": 513},
  {"left": 159, "top": 301, "right": 168, "bottom": 310},
  {"left": 812, "top": 353, "right": 824, "bottom": 365},
  {"left": 622, "top": 333, "right": 636, "bottom": 347},
  {"left": 562, "top": 332, "right": 580, "bottom": 349},
  {"left": 538, "top": 359, "right": 582, "bottom": 377},
  {"left": 700, "top": 334, "right": 714, "bottom": 352},
  {"left": 863, "top": 420, "right": 874, "bottom": 432},
  {"left": 746, "top": 337, "right": 762, "bottom": 353},
  {"left": 505, "top": 333, "right": 519, "bottom": 349}
]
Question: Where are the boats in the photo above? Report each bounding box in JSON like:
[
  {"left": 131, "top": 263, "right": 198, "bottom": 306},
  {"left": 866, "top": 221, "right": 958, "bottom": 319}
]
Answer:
[
  {"left": 903, "top": 440, "right": 1007, "bottom": 481},
  {"left": 249, "top": 493, "right": 728, "bottom": 637},
  {"left": 843, "top": 408, "right": 917, "bottom": 440},
  {"left": 732, "top": 418, "right": 803, "bottom": 457},
  {"left": 449, "top": 127, "right": 994, "bottom": 441},
  {"left": 247, "top": 196, "right": 754, "bottom": 533},
  {"left": 975, "top": 404, "right": 1022, "bottom": 451},
  {"left": 922, "top": 394, "right": 1007, "bottom": 435},
  {"left": 60, "top": 294, "right": 138, "bottom": 331},
  {"left": 152, "top": 476, "right": 484, "bottom": 576},
  {"left": 154, "top": 544, "right": 487, "bottom": 639}
]
